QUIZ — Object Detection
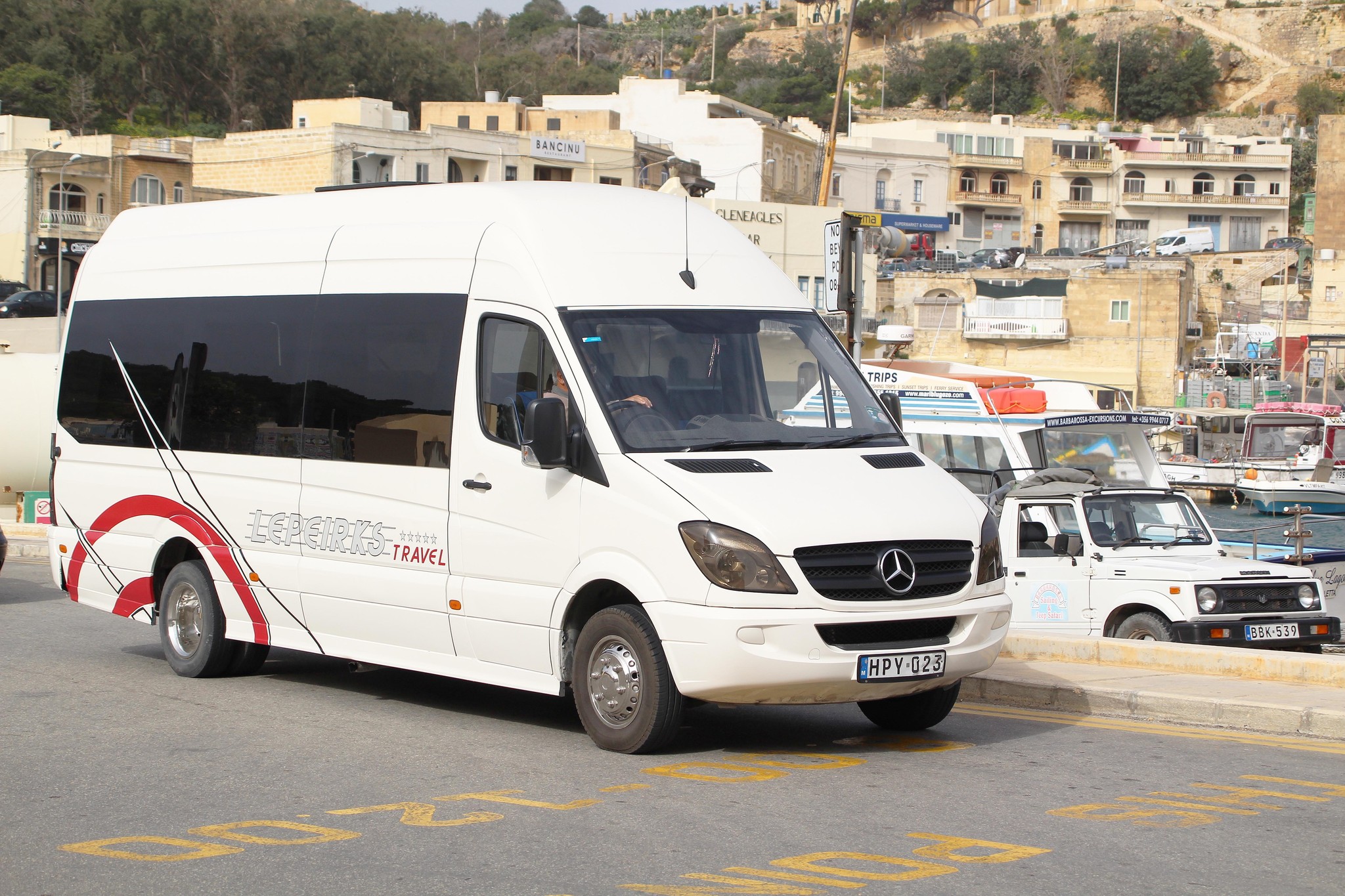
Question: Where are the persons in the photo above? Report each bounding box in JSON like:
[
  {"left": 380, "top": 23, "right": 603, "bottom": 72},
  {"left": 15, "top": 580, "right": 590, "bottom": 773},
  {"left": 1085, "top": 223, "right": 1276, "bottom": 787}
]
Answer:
[{"left": 544, "top": 345, "right": 653, "bottom": 434}]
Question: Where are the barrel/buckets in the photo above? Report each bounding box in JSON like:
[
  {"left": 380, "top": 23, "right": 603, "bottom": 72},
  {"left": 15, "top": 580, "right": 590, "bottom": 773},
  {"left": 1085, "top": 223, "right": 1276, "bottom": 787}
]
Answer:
[
  {"left": 664, "top": 68, "right": 671, "bottom": 79},
  {"left": 1247, "top": 342, "right": 1259, "bottom": 358},
  {"left": 484, "top": 91, "right": 500, "bottom": 102},
  {"left": 507, "top": 96, "right": 522, "bottom": 104}
]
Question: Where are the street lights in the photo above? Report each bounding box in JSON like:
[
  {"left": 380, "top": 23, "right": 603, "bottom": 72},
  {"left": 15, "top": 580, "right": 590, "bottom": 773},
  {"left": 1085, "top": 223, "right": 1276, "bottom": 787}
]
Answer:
[{"left": 55, "top": 153, "right": 83, "bottom": 352}]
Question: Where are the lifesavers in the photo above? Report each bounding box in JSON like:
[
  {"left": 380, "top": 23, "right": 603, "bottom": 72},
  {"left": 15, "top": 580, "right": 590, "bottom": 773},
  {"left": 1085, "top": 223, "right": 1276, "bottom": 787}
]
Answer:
[{"left": 1207, "top": 391, "right": 1226, "bottom": 409}]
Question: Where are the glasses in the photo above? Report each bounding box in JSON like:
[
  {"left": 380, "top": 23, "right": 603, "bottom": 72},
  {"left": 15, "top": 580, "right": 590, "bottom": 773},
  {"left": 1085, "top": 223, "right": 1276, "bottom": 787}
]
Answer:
[{"left": 589, "top": 364, "right": 597, "bottom": 374}]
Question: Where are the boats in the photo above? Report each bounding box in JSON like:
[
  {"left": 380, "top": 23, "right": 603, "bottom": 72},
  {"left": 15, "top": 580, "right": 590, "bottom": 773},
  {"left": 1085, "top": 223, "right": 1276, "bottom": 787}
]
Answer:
[
  {"left": 775, "top": 354, "right": 1345, "bottom": 648},
  {"left": 1196, "top": 323, "right": 1277, "bottom": 376},
  {"left": 1114, "top": 401, "right": 1300, "bottom": 502},
  {"left": 1232, "top": 404, "right": 1345, "bottom": 519}
]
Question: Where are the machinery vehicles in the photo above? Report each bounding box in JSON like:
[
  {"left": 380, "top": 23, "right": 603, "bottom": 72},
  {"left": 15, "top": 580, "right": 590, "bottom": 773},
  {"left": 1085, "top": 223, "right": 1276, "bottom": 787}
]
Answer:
[{"left": 871, "top": 225, "right": 935, "bottom": 266}]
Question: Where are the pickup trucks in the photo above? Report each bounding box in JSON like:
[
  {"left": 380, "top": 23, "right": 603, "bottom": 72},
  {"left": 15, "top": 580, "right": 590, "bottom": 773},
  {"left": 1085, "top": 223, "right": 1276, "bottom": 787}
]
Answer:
[{"left": 944, "top": 461, "right": 1342, "bottom": 654}]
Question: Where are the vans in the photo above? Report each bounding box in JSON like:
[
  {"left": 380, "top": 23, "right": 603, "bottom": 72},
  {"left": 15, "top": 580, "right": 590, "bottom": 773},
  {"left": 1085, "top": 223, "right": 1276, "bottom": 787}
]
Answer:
[
  {"left": 49, "top": 180, "right": 1014, "bottom": 758},
  {"left": 0, "top": 280, "right": 30, "bottom": 302},
  {"left": 1145, "top": 227, "right": 1215, "bottom": 256}
]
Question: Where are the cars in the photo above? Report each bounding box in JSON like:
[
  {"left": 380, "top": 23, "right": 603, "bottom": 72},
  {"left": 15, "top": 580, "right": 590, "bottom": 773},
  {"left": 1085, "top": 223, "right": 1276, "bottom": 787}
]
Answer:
[
  {"left": 0, "top": 290, "right": 66, "bottom": 319},
  {"left": 1134, "top": 246, "right": 1150, "bottom": 256},
  {"left": 932, "top": 247, "right": 1009, "bottom": 269},
  {"left": 1043, "top": 247, "right": 1074, "bottom": 257},
  {"left": 882, "top": 260, "right": 939, "bottom": 273},
  {"left": 1009, "top": 246, "right": 1043, "bottom": 262},
  {"left": 1264, "top": 237, "right": 1306, "bottom": 251}
]
